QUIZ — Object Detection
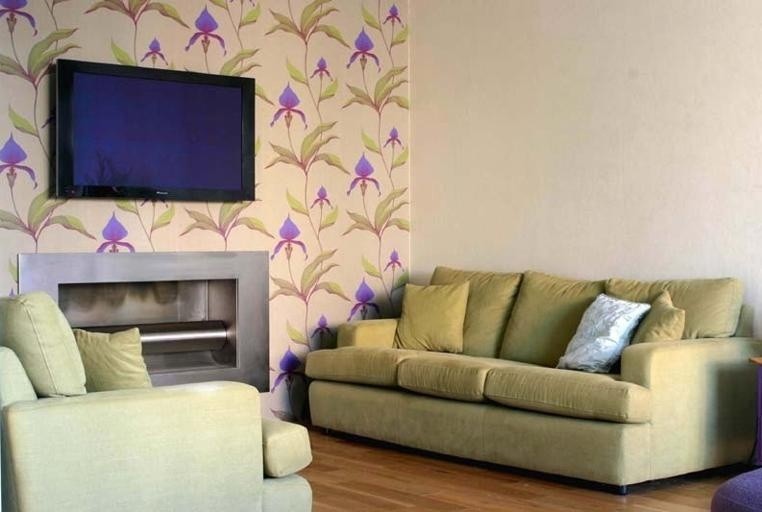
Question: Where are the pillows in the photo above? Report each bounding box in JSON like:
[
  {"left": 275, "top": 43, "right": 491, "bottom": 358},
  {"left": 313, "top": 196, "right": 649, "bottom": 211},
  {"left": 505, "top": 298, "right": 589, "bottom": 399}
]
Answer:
[
  {"left": 393, "top": 280, "right": 470, "bottom": 353},
  {"left": 71, "top": 325, "right": 153, "bottom": 393},
  {"left": 557, "top": 290, "right": 685, "bottom": 374}
]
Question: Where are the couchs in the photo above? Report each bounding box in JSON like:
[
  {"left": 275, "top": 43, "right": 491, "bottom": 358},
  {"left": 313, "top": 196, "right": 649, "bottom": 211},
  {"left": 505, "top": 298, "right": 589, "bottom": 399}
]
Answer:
[
  {"left": 1, "top": 292, "right": 315, "bottom": 511},
  {"left": 304, "top": 265, "right": 759, "bottom": 494}
]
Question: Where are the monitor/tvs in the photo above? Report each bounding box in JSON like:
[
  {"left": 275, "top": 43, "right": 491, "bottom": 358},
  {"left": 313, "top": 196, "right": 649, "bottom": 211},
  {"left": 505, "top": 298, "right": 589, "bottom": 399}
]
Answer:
[{"left": 56, "top": 58, "right": 255, "bottom": 202}]
